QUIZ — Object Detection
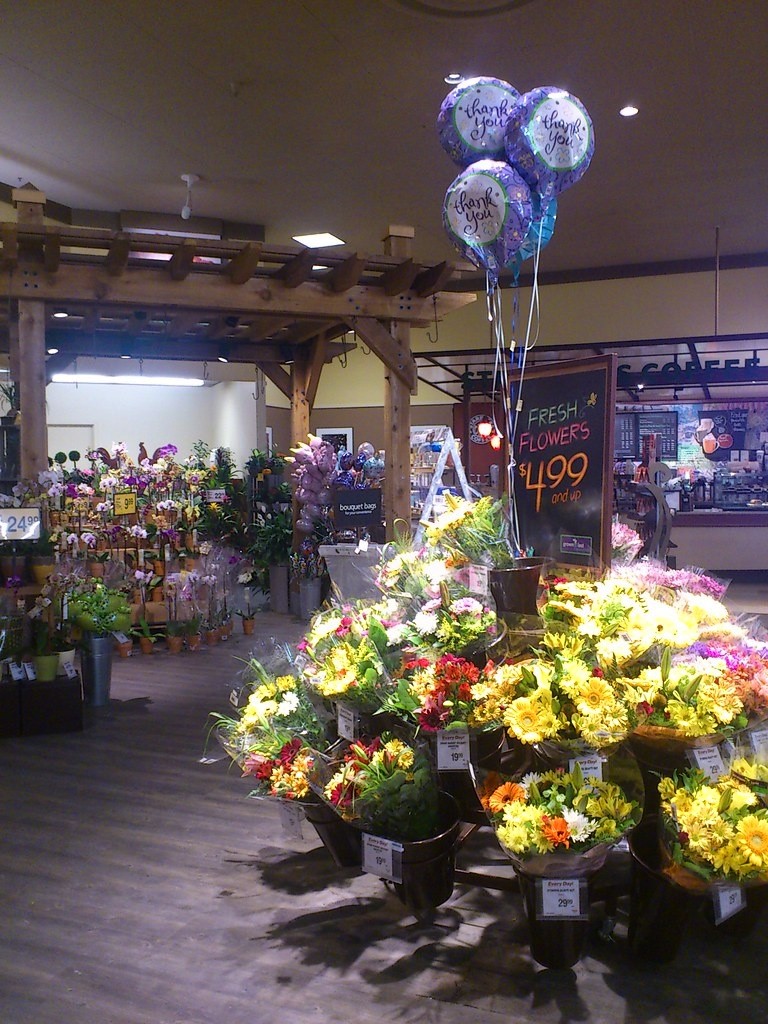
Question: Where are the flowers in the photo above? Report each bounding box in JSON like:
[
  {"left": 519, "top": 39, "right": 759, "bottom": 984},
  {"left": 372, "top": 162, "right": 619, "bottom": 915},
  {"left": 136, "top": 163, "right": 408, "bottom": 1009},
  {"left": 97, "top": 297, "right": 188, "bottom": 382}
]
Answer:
[
  {"left": 645, "top": 756, "right": 768, "bottom": 892},
  {"left": 203, "top": 660, "right": 440, "bottom": 841},
  {"left": 296, "top": 496, "right": 768, "bottom": 746},
  {"left": 484, "top": 760, "right": 640, "bottom": 880},
  {"left": 0, "top": 443, "right": 289, "bottom": 654}
]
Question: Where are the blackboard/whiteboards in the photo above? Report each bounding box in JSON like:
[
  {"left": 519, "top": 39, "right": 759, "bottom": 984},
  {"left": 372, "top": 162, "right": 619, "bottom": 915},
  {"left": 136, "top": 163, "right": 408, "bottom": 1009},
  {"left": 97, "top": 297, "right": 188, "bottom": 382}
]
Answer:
[{"left": 504, "top": 350, "right": 619, "bottom": 572}]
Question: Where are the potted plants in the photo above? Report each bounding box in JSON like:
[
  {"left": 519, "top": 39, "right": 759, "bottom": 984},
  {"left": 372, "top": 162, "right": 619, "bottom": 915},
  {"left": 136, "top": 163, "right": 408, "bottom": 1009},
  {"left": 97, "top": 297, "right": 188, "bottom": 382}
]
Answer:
[{"left": 253, "top": 509, "right": 294, "bottom": 614}]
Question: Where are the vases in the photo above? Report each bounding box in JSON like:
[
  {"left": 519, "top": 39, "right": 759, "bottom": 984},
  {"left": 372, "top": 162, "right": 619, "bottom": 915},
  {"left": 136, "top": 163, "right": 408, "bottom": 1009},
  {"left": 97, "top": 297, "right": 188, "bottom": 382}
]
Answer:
[
  {"left": 206, "top": 628, "right": 218, "bottom": 646},
  {"left": 217, "top": 624, "right": 231, "bottom": 640},
  {"left": 186, "top": 634, "right": 201, "bottom": 650},
  {"left": 168, "top": 638, "right": 183, "bottom": 654},
  {"left": 140, "top": 637, "right": 154, "bottom": 655},
  {"left": 48, "top": 510, "right": 70, "bottom": 528},
  {"left": 126, "top": 513, "right": 139, "bottom": 525},
  {"left": 182, "top": 509, "right": 197, "bottom": 525},
  {"left": 186, "top": 558, "right": 200, "bottom": 572},
  {"left": 197, "top": 587, "right": 210, "bottom": 600},
  {"left": 130, "top": 589, "right": 146, "bottom": 604},
  {"left": 144, "top": 510, "right": 155, "bottom": 525},
  {"left": 295, "top": 554, "right": 768, "bottom": 968},
  {"left": 86, "top": 562, "right": 105, "bottom": 577},
  {"left": 243, "top": 620, "right": 255, "bottom": 635},
  {"left": 162, "top": 510, "right": 177, "bottom": 525},
  {"left": 132, "top": 561, "right": 147, "bottom": 574},
  {"left": 169, "top": 560, "right": 182, "bottom": 572},
  {"left": 151, "top": 587, "right": 163, "bottom": 602},
  {"left": 300, "top": 577, "right": 322, "bottom": 622},
  {"left": 31, "top": 555, "right": 56, "bottom": 587},
  {"left": 117, "top": 640, "right": 132, "bottom": 656},
  {"left": 35, "top": 655, "right": 57, "bottom": 681},
  {"left": 154, "top": 560, "right": 165, "bottom": 576},
  {"left": 81, "top": 639, "right": 113, "bottom": 707},
  {"left": 53, "top": 648, "right": 76, "bottom": 675},
  {"left": 93, "top": 497, "right": 105, "bottom": 511},
  {"left": 1, "top": 555, "right": 25, "bottom": 584}
]
